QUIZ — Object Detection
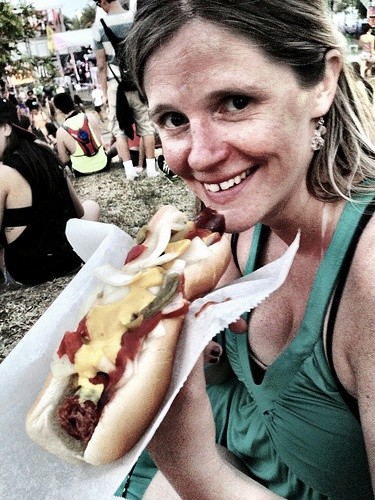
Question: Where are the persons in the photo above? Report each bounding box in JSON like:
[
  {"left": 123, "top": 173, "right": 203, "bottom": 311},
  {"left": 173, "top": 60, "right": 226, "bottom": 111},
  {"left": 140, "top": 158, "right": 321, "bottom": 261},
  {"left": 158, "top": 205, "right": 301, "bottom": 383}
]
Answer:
[
  {"left": 0, "top": 97, "right": 99, "bottom": 285},
  {"left": 0, "top": 0, "right": 179, "bottom": 181},
  {"left": 358, "top": 23, "right": 375, "bottom": 77},
  {"left": 125, "top": 0, "right": 375, "bottom": 500}
]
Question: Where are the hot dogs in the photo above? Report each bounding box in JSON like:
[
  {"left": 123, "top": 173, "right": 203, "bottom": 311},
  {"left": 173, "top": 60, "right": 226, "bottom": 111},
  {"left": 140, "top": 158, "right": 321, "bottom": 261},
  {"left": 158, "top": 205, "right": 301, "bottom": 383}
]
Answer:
[{"left": 25, "top": 206, "right": 233, "bottom": 467}]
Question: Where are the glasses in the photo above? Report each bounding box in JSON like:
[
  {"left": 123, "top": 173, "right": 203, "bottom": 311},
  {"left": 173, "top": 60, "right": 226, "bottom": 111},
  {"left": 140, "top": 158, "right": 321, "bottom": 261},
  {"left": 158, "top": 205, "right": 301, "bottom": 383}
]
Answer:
[{"left": 96, "top": 0, "right": 101, "bottom": 6}]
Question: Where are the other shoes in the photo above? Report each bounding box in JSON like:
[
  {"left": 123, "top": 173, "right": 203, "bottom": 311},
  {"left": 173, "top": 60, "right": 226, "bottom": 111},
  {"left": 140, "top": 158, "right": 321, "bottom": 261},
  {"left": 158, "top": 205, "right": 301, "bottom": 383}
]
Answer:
[{"left": 158, "top": 155, "right": 177, "bottom": 183}]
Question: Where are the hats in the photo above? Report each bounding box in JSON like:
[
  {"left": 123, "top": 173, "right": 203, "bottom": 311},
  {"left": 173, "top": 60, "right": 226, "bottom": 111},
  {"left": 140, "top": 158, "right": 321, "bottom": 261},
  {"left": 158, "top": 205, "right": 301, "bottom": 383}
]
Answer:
[{"left": 0, "top": 97, "right": 38, "bottom": 142}]
